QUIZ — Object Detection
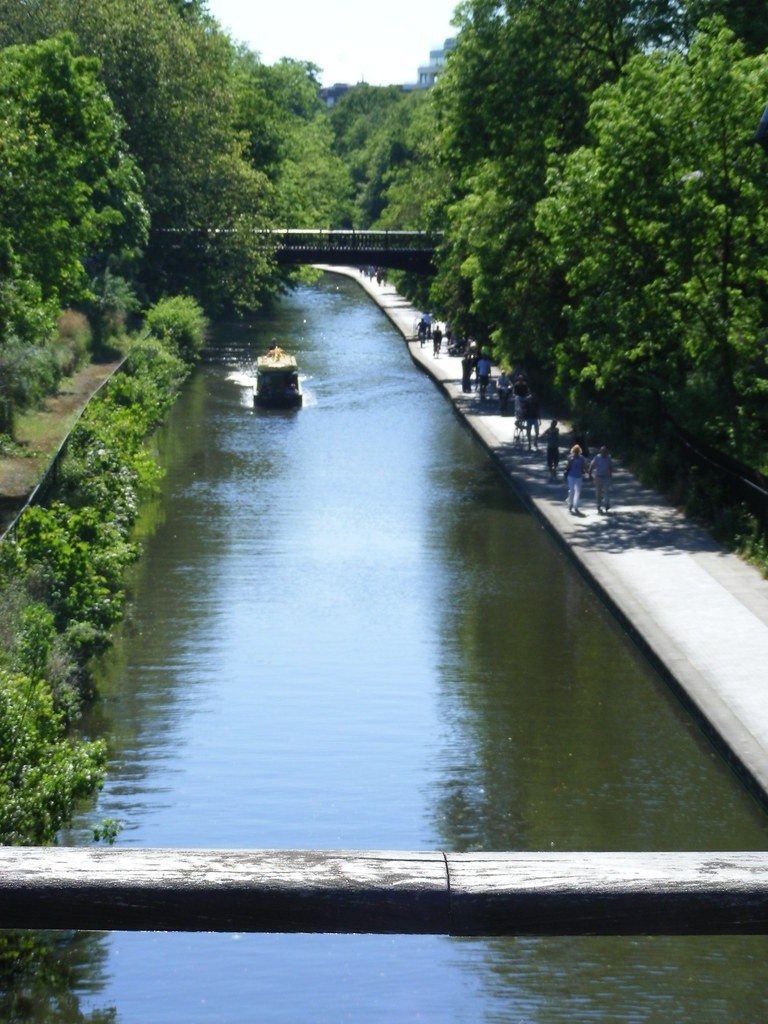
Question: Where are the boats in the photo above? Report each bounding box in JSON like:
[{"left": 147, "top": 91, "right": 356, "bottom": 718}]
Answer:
[{"left": 253, "top": 352, "right": 304, "bottom": 407}]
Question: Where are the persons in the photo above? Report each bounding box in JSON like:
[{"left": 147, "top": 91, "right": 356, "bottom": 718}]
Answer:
[
  {"left": 433, "top": 326, "right": 442, "bottom": 352},
  {"left": 542, "top": 419, "right": 560, "bottom": 472},
  {"left": 463, "top": 351, "right": 491, "bottom": 395},
  {"left": 564, "top": 445, "right": 591, "bottom": 512},
  {"left": 416, "top": 319, "right": 427, "bottom": 343},
  {"left": 589, "top": 446, "right": 613, "bottom": 512},
  {"left": 513, "top": 375, "right": 541, "bottom": 452},
  {"left": 496, "top": 370, "right": 513, "bottom": 410},
  {"left": 445, "top": 322, "right": 454, "bottom": 345},
  {"left": 359, "top": 265, "right": 388, "bottom": 287},
  {"left": 423, "top": 312, "right": 431, "bottom": 337},
  {"left": 448, "top": 338, "right": 470, "bottom": 357}
]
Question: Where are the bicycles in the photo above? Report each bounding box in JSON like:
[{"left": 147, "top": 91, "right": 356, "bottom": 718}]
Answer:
[{"left": 512, "top": 418, "right": 526, "bottom": 451}]
